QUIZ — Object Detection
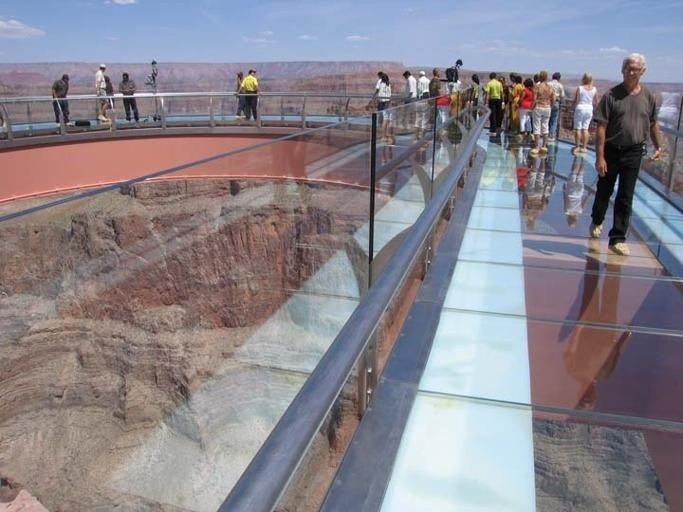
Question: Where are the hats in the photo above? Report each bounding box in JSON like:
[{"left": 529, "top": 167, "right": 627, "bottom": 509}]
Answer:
[
  {"left": 248, "top": 69, "right": 257, "bottom": 74},
  {"left": 99, "top": 63, "right": 106, "bottom": 70}
]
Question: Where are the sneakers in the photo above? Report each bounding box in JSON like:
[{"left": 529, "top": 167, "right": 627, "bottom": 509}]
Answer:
[
  {"left": 485, "top": 131, "right": 497, "bottom": 138},
  {"left": 608, "top": 241, "right": 630, "bottom": 257},
  {"left": 506, "top": 128, "right": 556, "bottom": 143},
  {"left": 380, "top": 126, "right": 450, "bottom": 150},
  {"left": 56, "top": 121, "right": 73, "bottom": 127},
  {"left": 531, "top": 146, "right": 548, "bottom": 154},
  {"left": 97, "top": 113, "right": 112, "bottom": 122},
  {"left": 590, "top": 220, "right": 602, "bottom": 238},
  {"left": 571, "top": 146, "right": 588, "bottom": 152}
]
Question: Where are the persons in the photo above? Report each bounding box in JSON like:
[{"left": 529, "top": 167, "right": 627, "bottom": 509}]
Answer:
[
  {"left": 565, "top": 254, "right": 633, "bottom": 412},
  {"left": 235, "top": 72, "right": 244, "bottom": 117},
  {"left": 118, "top": 72, "right": 140, "bottom": 123},
  {"left": 589, "top": 53, "right": 664, "bottom": 257},
  {"left": 96, "top": 75, "right": 114, "bottom": 121},
  {"left": 234, "top": 69, "right": 261, "bottom": 120},
  {"left": 95, "top": 64, "right": 108, "bottom": 122},
  {"left": 372, "top": 59, "right": 599, "bottom": 155},
  {"left": 52, "top": 74, "right": 75, "bottom": 126},
  {"left": 376, "top": 142, "right": 593, "bottom": 230}
]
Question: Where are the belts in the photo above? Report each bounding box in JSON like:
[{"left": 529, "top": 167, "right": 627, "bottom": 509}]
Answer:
[{"left": 605, "top": 142, "right": 645, "bottom": 151}]
